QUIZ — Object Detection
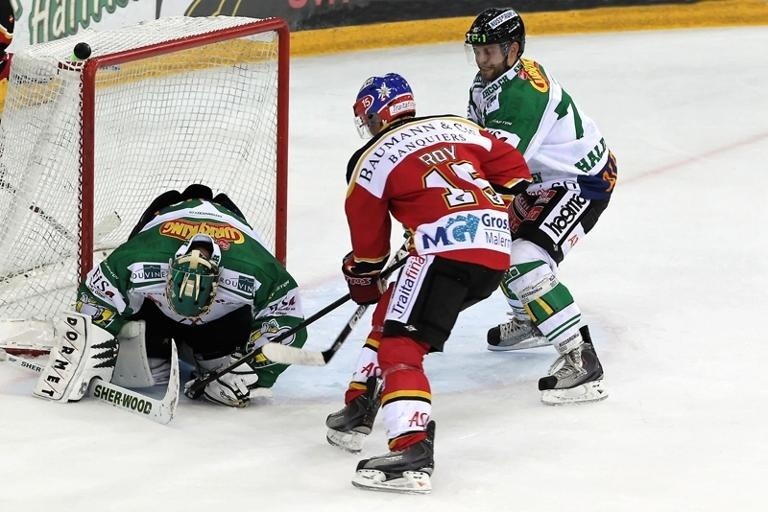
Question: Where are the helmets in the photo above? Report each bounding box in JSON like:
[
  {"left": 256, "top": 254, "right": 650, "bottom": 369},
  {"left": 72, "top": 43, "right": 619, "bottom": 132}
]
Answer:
[
  {"left": 165, "top": 250, "right": 218, "bottom": 319},
  {"left": 463, "top": 7, "right": 526, "bottom": 66},
  {"left": 352, "top": 73, "right": 416, "bottom": 140}
]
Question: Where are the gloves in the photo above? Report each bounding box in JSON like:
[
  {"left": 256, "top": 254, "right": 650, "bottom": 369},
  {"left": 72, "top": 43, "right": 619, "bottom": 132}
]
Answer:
[{"left": 341, "top": 249, "right": 391, "bottom": 308}]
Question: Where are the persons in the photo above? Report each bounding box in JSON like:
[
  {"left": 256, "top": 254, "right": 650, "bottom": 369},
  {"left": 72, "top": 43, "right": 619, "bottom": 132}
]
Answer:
[
  {"left": 326, "top": 72, "right": 533, "bottom": 479},
  {"left": 32, "top": 184, "right": 308, "bottom": 407},
  {"left": 465, "top": 7, "right": 616, "bottom": 392}
]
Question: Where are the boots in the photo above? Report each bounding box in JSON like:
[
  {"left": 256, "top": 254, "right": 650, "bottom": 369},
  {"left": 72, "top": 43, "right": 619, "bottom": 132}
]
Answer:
[
  {"left": 324, "top": 374, "right": 386, "bottom": 438},
  {"left": 486, "top": 311, "right": 541, "bottom": 348},
  {"left": 355, "top": 417, "right": 437, "bottom": 479},
  {"left": 538, "top": 321, "right": 605, "bottom": 392}
]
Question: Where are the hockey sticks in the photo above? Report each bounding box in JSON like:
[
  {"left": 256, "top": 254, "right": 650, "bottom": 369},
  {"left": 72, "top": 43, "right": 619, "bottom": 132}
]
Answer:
[
  {"left": 184, "top": 236, "right": 411, "bottom": 399},
  {"left": 0, "top": 337, "right": 179, "bottom": 424},
  {"left": 1, "top": 174, "right": 121, "bottom": 252}
]
